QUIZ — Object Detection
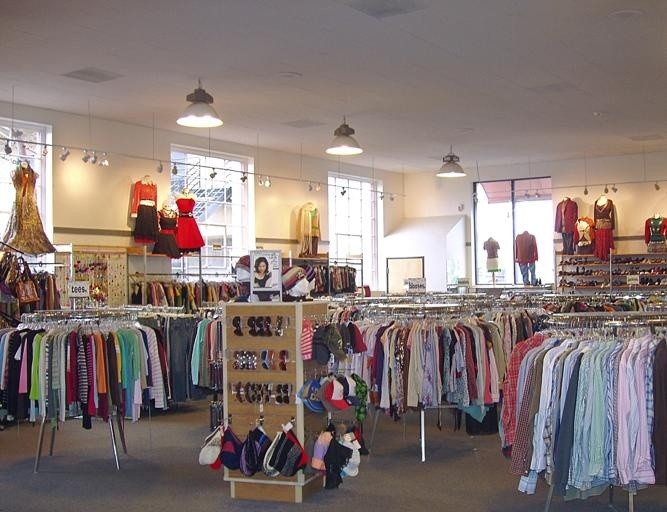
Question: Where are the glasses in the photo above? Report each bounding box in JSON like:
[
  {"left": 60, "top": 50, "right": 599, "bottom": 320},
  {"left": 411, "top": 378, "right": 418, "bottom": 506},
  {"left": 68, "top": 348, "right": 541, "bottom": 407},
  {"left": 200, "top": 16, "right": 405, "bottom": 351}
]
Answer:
[
  {"left": 232, "top": 349, "right": 276, "bottom": 370},
  {"left": 279, "top": 350, "right": 288, "bottom": 371},
  {"left": 233, "top": 316, "right": 244, "bottom": 336},
  {"left": 237, "top": 381, "right": 289, "bottom": 405},
  {"left": 247, "top": 316, "right": 284, "bottom": 337}
]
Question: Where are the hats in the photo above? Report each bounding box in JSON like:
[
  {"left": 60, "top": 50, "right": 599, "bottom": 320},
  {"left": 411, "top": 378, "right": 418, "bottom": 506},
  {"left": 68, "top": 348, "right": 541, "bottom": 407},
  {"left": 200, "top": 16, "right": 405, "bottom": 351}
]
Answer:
[
  {"left": 198, "top": 425, "right": 307, "bottom": 478},
  {"left": 311, "top": 425, "right": 369, "bottom": 488},
  {"left": 299, "top": 373, "right": 369, "bottom": 420},
  {"left": 300, "top": 319, "right": 368, "bottom": 366}
]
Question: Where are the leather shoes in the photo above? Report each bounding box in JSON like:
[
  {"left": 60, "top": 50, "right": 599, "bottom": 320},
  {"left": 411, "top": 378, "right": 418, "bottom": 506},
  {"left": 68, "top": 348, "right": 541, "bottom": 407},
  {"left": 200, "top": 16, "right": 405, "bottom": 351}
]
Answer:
[
  {"left": 612, "top": 255, "right": 667, "bottom": 286},
  {"left": 558, "top": 258, "right": 610, "bottom": 286}
]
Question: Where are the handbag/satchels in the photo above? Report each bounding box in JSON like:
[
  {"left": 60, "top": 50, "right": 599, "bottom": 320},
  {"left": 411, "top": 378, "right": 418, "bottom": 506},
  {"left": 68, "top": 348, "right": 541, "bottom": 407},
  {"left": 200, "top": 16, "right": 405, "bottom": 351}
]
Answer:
[{"left": 1, "top": 252, "right": 41, "bottom": 304}]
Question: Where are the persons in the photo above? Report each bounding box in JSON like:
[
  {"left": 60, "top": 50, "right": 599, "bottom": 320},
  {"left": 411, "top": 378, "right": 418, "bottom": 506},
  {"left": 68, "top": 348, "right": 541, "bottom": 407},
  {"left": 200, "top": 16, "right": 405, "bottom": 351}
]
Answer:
[
  {"left": 516, "top": 232, "right": 538, "bottom": 285},
  {"left": 483, "top": 237, "right": 500, "bottom": 270},
  {"left": 554, "top": 197, "right": 578, "bottom": 254},
  {"left": 297, "top": 203, "right": 321, "bottom": 256},
  {"left": 645, "top": 214, "right": 667, "bottom": 253},
  {"left": 152, "top": 200, "right": 180, "bottom": 258},
  {"left": 253, "top": 257, "right": 272, "bottom": 288},
  {"left": 175, "top": 188, "right": 205, "bottom": 250},
  {"left": 130, "top": 175, "right": 158, "bottom": 243},
  {"left": 594, "top": 196, "right": 615, "bottom": 259}
]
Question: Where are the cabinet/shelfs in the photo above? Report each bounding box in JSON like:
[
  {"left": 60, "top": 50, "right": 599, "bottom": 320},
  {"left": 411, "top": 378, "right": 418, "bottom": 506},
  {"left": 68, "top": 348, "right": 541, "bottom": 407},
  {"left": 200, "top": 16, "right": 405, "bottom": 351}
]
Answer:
[
  {"left": 554, "top": 248, "right": 667, "bottom": 294},
  {"left": 221, "top": 299, "right": 329, "bottom": 503}
]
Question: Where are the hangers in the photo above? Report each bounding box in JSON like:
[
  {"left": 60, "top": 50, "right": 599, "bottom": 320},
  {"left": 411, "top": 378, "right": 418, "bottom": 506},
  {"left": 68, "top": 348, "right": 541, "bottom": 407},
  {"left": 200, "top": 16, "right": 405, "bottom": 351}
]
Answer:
[
  {"left": 16, "top": 273, "right": 238, "bottom": 335},
  {"left": 331, "top": 292, "right": 666, "bottom": 343}
]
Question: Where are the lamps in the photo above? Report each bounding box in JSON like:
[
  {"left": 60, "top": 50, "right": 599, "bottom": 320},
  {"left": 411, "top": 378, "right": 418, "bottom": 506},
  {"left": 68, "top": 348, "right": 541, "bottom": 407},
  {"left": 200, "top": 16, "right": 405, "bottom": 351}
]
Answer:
[
  {"left": 91, "top": 152, "right": 97, "bottom": 164},
  {"left": 380, "top": 193, "right": 384, "bottom": 200},
  {"left": 604, "top": 184, "right": 608, "bottom": 193},
  {"left": 157, "top": 161, "right": 163, "bottom": 173},
  {"left": 82, "top": 149, "right": 91, "bottom": 162},
  {"left": 258, "top": 175, "right": 264, "bottom": 185},
  {"left": 655, "top": 183, "right": 660, "bottom": 190},
  {"left": 240, "top": 173, "right": 247, "bottom": 183},
  {"left": 175, "top": 78, "right": 224, "bottom": 129},
  {"left": 316, "top": 183, "right": 320, "bottom": 191},
  {"left": 99, "top": 153, "right": 109, "bottom": 166},
  {"left": 5, "top": 141, "right": 12, "bottom": 154},
  {"left": 584, "top": 188, "right": 588, "bottom": 195},
  {"left": 325, "top": 115, "right": 363, "bottom": 157},
  {"left": 308, "top": 182, "right": 313, "bottom": 191},
  {"left": 210, "top": 168, "right": 216, "bottom": 179},
  {"left": 611, "top": 185, "right": 617, "bottom": 193},
  {"left": 172, "top": 163, "right": 178, "bottom": 174},
  {"left": 265, "top": 176, "right": 272, "bottom": 187},
  {"left": 43, "top": 145, "right": 48, "bottom": 155},
  {"left": 525, "top": 193, "right": 529, "bottom": 200},
  {"left": 535, "top": 193, "right": 540, "bottom": 199},
  {"left": 341, "top": 188, "right": 346, "bottom": 196},
  {"left": 436, "top": 143, "right": 467, "bottom": 178},
  {"left": 60, "top": 147, "right": 69, "bottom": 161}
]
